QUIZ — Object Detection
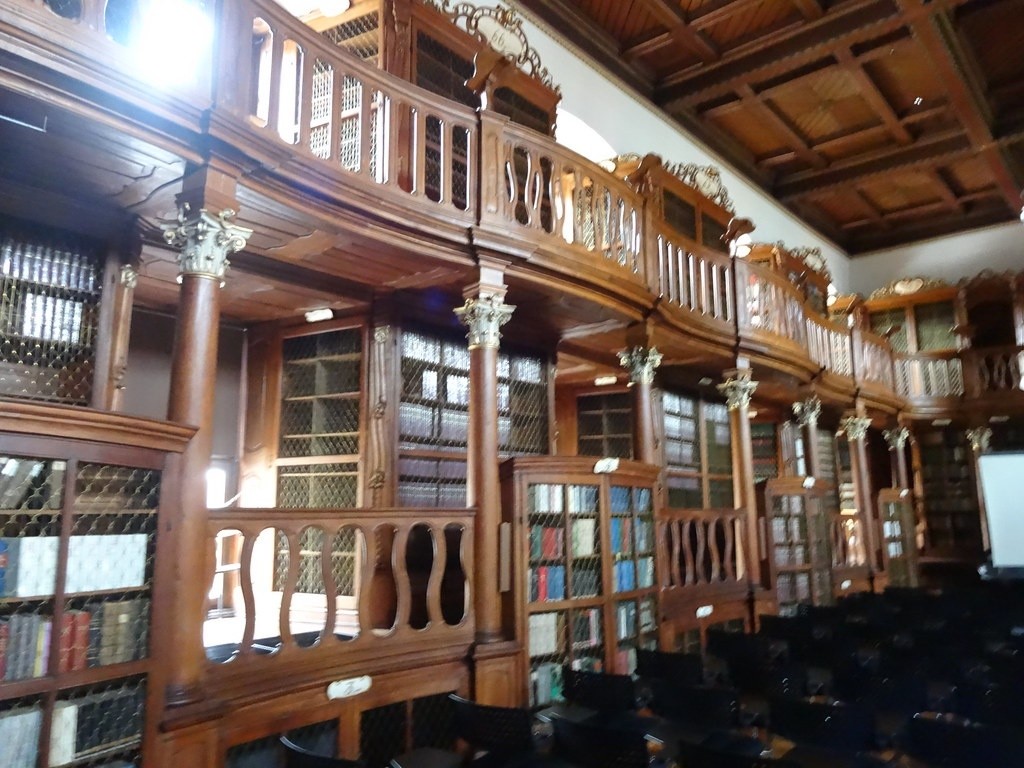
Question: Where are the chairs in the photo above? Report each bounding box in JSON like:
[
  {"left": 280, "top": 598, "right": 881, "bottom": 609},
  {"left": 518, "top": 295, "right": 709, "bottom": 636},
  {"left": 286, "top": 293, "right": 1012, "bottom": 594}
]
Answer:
[{"left": 279, "top": 578, "right": 1024, "bottom": 768}]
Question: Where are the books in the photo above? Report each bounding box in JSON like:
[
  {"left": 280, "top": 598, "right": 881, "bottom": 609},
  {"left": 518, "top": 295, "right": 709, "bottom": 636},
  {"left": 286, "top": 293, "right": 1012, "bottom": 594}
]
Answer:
[
  {"left": 0, "top": 457, "right": 150, "bottom": 514},
  {"left": 0, "top": 238, "right": 99, "bottom": 348},
  {"left": 392, "top": 331, "right": 863, "bottom": 707},
  {"left": 0, "top": 598, "right": 152, "bottom": 682},
  {"left": 0, "top": 685, "right": 144, "bottom": 768}
]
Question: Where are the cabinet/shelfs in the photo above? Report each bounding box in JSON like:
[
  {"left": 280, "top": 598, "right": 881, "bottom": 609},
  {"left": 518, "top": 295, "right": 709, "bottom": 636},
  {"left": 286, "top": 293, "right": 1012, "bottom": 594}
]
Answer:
[
  {"left": 577, "top": 393, "right": 632, "bottom": 460},
  {"left": 0, "top": 174, "right": 144, "bottom": 415},
  {"left": 278, "top": 312, "right": 556, "bottom": 631},
  {"left": 500, "top": 454, "right": 666, "bottom": 708},
  {"left": 297, "top": 0, "right": 550, "bottom": 231},
  {"left": 0, "top": 394, "right": 200, "bottom": 768},
  {"left": 859, "top": 275, "right": 973, "bottom": 402}
]
